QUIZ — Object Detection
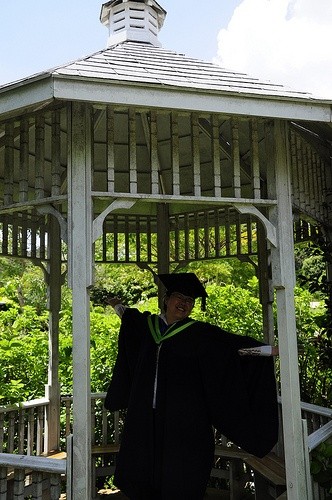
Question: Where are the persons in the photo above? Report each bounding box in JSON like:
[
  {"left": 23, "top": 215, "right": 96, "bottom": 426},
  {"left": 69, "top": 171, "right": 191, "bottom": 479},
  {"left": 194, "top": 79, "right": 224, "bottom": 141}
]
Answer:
[{"left": 104, "top": 272, "right": 279, "bottom": 499}]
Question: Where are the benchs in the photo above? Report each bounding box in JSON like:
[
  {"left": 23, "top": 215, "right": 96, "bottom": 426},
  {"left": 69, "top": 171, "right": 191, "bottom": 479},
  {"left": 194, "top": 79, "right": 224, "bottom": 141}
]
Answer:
[{"left": 7, "top": 445, "right": 287, "bottom": 500}]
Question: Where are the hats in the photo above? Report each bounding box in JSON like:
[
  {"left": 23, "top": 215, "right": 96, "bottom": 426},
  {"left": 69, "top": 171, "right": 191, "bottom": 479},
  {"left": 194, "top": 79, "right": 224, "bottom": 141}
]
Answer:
[{"left": 158, "top": 273, "right": 208, "bottom": 311}]
